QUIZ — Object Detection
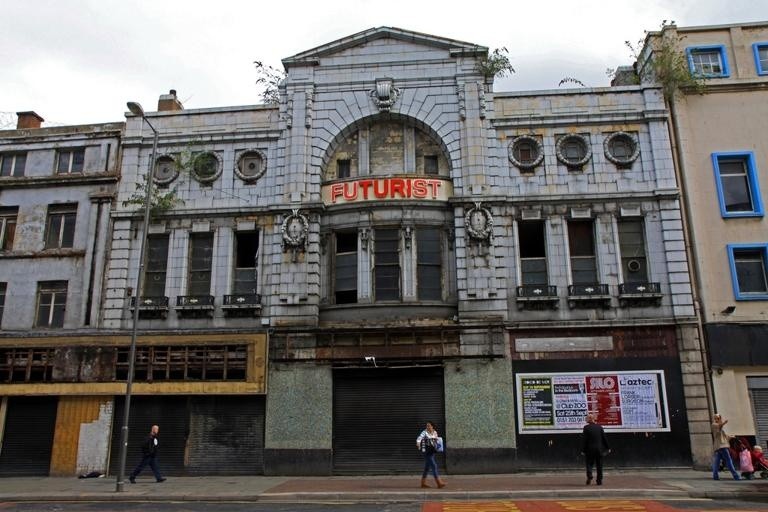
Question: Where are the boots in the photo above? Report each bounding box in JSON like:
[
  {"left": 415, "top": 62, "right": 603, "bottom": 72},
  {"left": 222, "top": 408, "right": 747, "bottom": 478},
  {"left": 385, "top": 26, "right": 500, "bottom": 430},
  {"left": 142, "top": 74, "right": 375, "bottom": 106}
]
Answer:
[
  {"left": 434, "top": 477, "right": 449, "bottom": 489},
  {"left": 420, "top": 477, "right": 433, "bottom": 488}
]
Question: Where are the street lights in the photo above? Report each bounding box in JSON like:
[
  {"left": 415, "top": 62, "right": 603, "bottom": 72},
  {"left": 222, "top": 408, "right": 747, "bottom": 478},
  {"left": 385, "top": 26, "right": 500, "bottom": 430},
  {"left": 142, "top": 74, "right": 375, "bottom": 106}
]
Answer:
[{"left": 116, "top": 100, "right": 160, "bottom": 491}]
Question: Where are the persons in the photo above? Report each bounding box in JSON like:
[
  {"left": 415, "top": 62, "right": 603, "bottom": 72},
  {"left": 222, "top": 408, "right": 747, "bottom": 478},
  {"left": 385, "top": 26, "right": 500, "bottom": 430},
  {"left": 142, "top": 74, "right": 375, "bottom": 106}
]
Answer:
[
  {"left": 752, "top": 445, "right": 767, "bottom": 468},
  {"left": 709, "top": 414, "right": 742, "bottom": 480},
  {"left": 580, "top": 413, "right": 611, "bottom": 485},
  {"left": 416, "top": 422, "right": 447, "bottom": 488},
  {"left": 129, "top": 424, "right": 167, "bottom": 484}
]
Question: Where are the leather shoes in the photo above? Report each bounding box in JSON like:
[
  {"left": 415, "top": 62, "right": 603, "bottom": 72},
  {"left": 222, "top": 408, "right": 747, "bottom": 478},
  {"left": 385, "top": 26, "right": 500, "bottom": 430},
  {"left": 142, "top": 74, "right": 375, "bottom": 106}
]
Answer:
[
  {"left": 128, "top": 475, "right": 136, "bottom": 483},
  {"left": 157, "top": 478, "right": 167, "bottom": 483},
  {"left": 586, "top": 480, "right": 601, "bottom": 485}
]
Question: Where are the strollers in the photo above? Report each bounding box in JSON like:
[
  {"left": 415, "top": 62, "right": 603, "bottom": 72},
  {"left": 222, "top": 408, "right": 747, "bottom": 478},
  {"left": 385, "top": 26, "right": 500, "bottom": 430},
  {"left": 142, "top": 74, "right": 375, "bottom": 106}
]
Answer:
[{"left": 730, "top": 436, "right": 768, "bottom": 479}]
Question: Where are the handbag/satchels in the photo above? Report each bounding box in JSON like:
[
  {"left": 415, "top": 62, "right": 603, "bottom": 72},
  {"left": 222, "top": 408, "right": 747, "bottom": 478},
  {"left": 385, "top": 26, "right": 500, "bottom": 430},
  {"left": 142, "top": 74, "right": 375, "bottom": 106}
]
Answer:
[
  {"left": 739, "top": 450, "right": 753, "bottom": 472},
  {"left": 420, "top": 437, "right": 443, "bottom": 454}
]
